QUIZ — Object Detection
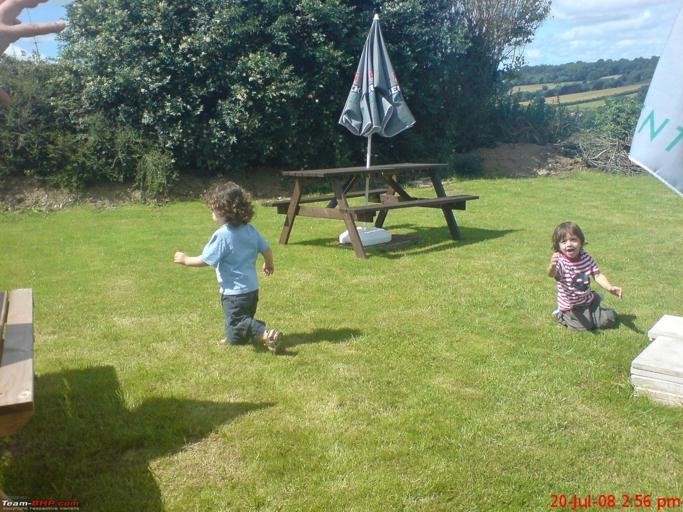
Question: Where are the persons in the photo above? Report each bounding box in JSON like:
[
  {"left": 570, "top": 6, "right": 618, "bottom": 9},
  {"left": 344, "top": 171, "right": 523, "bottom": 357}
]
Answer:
[
  {"left": 173, "top": 180, "right": 284, "bottom": 353},
  {"left": 546, "top": 219, "right": 624, "bottom": 330}
]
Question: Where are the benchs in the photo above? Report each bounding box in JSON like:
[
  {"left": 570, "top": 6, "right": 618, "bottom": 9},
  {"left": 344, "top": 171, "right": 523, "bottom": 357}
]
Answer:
[
  {"left": 0, "top": 288, "right": 37, "bottom": 438},
  {"left": 260, "top": 188, "right": 479, "bottom": 260}
]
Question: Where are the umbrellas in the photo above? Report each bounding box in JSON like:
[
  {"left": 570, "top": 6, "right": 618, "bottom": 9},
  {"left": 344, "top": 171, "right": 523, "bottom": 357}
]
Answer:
[
  {"left": 338, "top": 12, "right": 417, "bottom": 229},
  {"left": 627, "top": 6, "right": 683, "bottom": 199}
]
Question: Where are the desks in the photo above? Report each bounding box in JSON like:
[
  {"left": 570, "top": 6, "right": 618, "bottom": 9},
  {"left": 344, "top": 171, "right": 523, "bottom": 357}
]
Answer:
[{"left": 278, "top": 163, "right": 462, "bottom": 260}]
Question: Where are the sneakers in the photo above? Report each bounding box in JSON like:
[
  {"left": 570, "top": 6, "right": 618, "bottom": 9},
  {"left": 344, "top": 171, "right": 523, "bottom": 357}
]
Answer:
[{"left": 262, "top": 328, "right": 283, "bottom": 354}]
